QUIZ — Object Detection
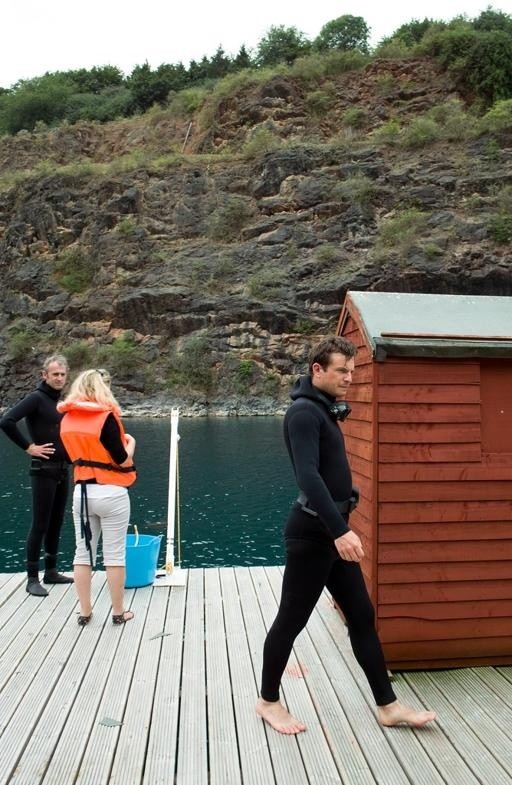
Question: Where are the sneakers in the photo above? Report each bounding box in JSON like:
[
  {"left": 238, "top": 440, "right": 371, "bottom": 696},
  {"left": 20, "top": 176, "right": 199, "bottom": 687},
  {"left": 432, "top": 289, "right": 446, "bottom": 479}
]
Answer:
[
  {"left": 44, "top": 568, "right": 74, "bottom": 583},
  {"left": 26, "top": 577, "right": 49, "bottom": 596}
]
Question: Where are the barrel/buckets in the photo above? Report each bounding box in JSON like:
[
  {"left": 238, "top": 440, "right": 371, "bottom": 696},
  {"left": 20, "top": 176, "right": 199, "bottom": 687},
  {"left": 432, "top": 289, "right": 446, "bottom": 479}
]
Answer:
[{"left": 124, "top": 531, "right": 162, "bottom": 590}]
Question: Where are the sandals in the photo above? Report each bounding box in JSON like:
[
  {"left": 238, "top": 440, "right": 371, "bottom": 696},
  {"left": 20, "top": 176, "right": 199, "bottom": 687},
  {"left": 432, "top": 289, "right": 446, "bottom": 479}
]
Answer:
[
  {"left": 112, "top": 611, "right": 134, "bottom": 624},
  {"left": 77, "top": 611, "right": 92, "bottom": 625}
]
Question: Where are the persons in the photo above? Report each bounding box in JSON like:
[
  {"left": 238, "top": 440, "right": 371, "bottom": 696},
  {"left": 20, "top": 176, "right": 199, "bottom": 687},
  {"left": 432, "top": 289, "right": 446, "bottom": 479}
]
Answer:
[
  {"left": 255, "top": 334, "right": 437, "bottom": 736},
  {"left": 2, "top": 353, "right": 73, "bottom": 597},
  {"left": 56, "top": 369, "right": 138, "bottom": 628}
]
